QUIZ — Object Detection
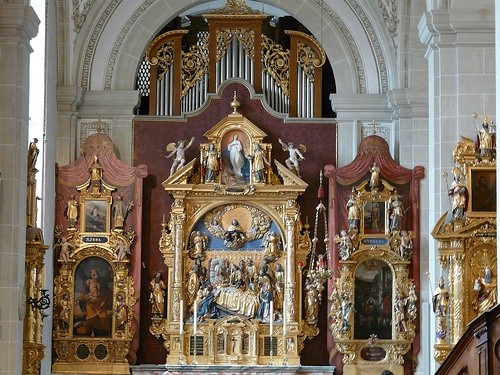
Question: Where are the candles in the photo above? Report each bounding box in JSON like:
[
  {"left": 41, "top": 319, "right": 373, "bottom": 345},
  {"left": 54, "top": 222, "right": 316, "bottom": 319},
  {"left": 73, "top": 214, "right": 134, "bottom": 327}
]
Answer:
[
  {"left": 41, "top": 265, "right": 47, "bottom": 289},
  {"left": 180, "top": 300, "right": 184, "bottom": 333},
  {"left": 193, "top": 300, "right": 198, "bottom": 333},
  {"left": 270, "top": 300, "right": 274, "bottom": 334},
  {"left": 283, "top": 301, "right": 287, "bottom": 333}
]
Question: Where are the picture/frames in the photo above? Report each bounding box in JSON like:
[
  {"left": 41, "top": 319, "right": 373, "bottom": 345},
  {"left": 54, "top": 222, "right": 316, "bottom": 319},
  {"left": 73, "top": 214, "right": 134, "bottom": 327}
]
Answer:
[
  {"left": 353, "top": 257, "right": 396, "bottom": 341},
  {"left": 360, "top": 192, "right": 391, "bottom": 237},
  {"left": 81, "top": 193, "right": 112, "bottom": 235},
  {"left": 467, "top": 166, "right": 497, "bottom": 218},
  {"left": 66, "top": 244, "right": 117, "bottom": 339}
]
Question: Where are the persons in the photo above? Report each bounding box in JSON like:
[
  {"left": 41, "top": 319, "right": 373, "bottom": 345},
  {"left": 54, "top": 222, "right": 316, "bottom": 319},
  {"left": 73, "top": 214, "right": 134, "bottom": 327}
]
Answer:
[
  {"left": 339, "top": 189, "right": 362, "bottom": 259},
  {"left": 473, "top": 266, "right": 496, "bottom": 316},
  {"left": 63, "top": 194, "right": 80, "bottom": 228},
  {"left": 56, "top": 237, "right": 76, "bottom": 264},
  {"left": 477, "top": 116, "right": 495, "bottom": 156},
  {"left": 113, "top": 195, "right": 129, "bottom": 228},
  {"left": 433, "top": 276, "right": 449, "bottom": 316},
  {"left": 225, "top": 219, "right": 245, "bottom": 243},
  {"left": 266, "top": 231, "right": 281, "bottom": 255},
  {"left": 227, "top": 134, "right": 245, "bottom": 177},
  {"left": 148, "top": 272, "right": 166, "bottom": 318},
  {"left": 79, "top": 269, "right": 111, "bottom": 338},
  {"left": 252, "top": 142, "right": 270, "bottom": 181},
  {"left": 388, "top": 196, "right": 414, "bottom": 259},
  {"left": 193, "top": 231, "right": 205, "bottom": 254},
  {"left": 278, "top": 138, "right": 306, "bottom": 178},
  {"left": 448, "top": 168, "right": 468, "bottom": 220},
  {"left": 114, "top": 241, "right": 129, "bottom": 262},
  {"left": 186, "top": 257, "right": 284, "bottom": 322},
  {"left": 205, "top": 143, "right": 220, "bottom": 183},
  {"left": 369, "top": 162, "right": 381, "bottom": 189},
  {"left": 167, "top": 137, "right": 196, "bottom": 176}
]
